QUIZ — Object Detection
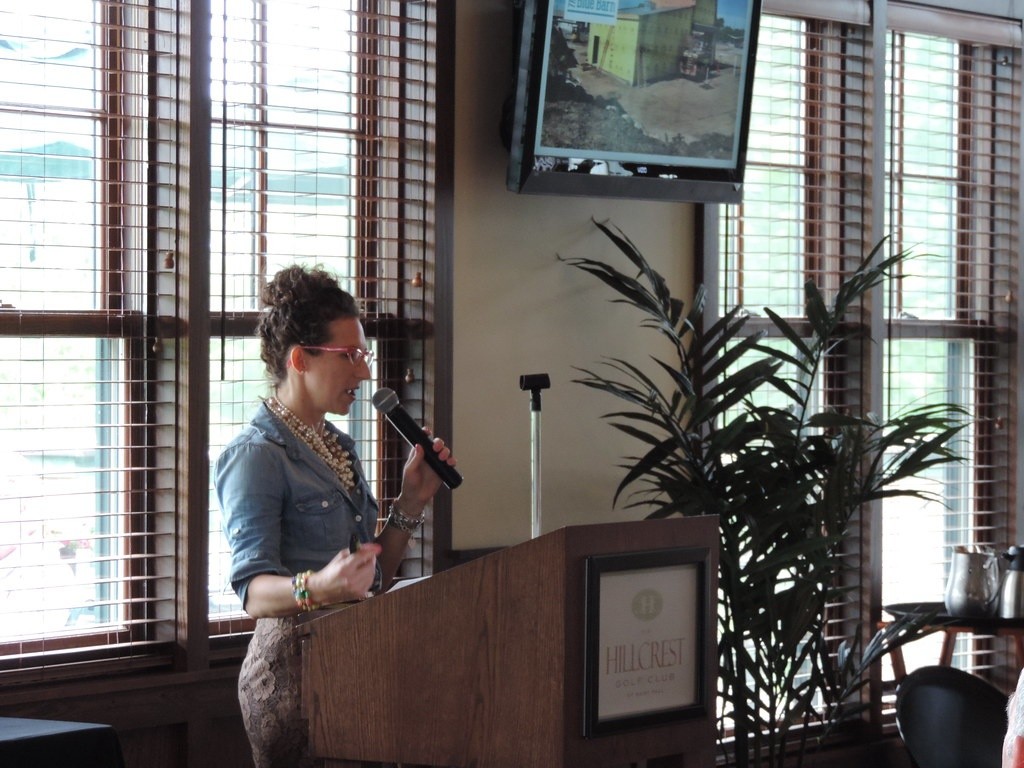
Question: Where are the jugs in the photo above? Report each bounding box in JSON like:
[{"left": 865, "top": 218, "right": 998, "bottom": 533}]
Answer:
[
  {"left": 999, "top": 545, "right": 1024, "bottom": 618},
  {"left": 944, "top": 552, "right": 999, "bottom": 616}
]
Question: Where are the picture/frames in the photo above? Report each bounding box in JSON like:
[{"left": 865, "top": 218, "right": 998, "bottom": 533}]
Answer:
[{"left": 579, "top": 547, "right": 713, "bottom": 738}]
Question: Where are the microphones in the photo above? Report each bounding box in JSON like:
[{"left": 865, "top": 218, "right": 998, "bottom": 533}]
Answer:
[{"left": 372, "top": 387, "right": 464, "bottom": 490}]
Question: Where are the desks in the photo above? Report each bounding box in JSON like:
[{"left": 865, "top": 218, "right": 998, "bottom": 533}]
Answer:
[{"left": 877, "top": 601, "right": 1024, "bottom": 695}]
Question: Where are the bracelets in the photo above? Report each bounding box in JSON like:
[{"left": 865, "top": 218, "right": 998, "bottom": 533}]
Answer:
[
  {"left": 388, "top": 500, "right": 425, "bottom": 535},
  {"left": 293, "top": 570, "right": 319, "bottom": 611}
]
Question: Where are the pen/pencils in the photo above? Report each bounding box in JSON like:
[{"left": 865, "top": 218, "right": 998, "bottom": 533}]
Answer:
[{"left": 349, "top": 532, "right": 361, "bottom": 554}]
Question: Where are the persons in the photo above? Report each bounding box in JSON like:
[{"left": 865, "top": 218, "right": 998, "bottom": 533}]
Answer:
[
  {"left": 1001, "top": 668, "right": 1024, "bottom": 768},
  {"left": 213, "top": 265, "right": 458, "bottom": 768}
]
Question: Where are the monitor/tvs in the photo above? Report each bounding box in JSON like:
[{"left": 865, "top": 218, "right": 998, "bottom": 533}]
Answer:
[{"left": 506, "top": 0, "right": 762, "bottom": 204}]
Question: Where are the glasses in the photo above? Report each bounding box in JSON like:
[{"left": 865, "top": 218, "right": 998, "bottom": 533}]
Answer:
[{"left": 287, "top": 344, "right": 374, "bottom": 368}]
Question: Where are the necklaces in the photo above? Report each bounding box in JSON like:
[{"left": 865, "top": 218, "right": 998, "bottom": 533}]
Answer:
[{"left": 264, "top": 397, "right": 354, "bottom": 490}]
